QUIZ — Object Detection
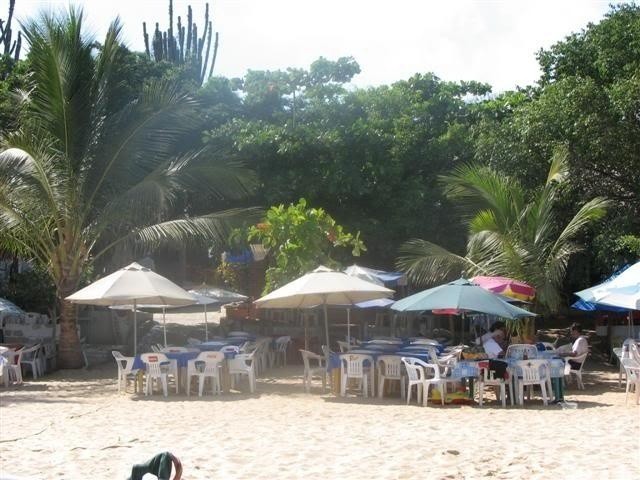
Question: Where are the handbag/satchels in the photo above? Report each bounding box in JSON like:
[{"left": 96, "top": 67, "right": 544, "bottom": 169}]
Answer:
[{"left": 480, "top": 360, "right": 508, "bottom": 379}]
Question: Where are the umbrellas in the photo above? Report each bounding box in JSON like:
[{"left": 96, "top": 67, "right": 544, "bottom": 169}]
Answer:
[
  {"left": 573, "top": 260, "right": 640, "bottom": 314},
  {"left": 307, "top": 296, "right": 397, "bottom": 351},
  {"left": 62, "top": 258, "right": 252, "bottom": 357},
  {"left": 252, "top": 263, "right": 398, "bottom": 394},
  {"left": 390, "top": 270, "right": 540, "bottom": 345},
  {"left": 429, "top": 274, "right": 536, "bottom": 331},
  {"left": 569, "top": 263, "right": 633, "bottom": 335},
  {"left": 343, "top": 264, "right": 406, "bottom": 282}
]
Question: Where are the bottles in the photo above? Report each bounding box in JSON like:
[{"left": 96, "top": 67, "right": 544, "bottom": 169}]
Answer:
[{"left": 523, "top": 348, "right": 529, "bottom": 361}]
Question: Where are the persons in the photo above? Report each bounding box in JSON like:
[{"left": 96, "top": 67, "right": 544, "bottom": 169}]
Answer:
[
  {"left": 481, "top": 329, "right": 508, "bottom": 380},
  {"left": 555, "top": 322, "right": 589, "bottom": 371},
  {"left": 475, "top": 321, "right": 506, "bottom": 347}
]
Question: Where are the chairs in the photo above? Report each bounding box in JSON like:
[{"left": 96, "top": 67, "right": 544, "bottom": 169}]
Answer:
[
  {"left": 612, "top": 335, "right": 640, "bottom": 405},
  {"left": 1, "top": 341, "right": 44, "bottom": 390},
  {"left": 298, "top": 333, "right": 587, "bottom": 409},
  {"left": 111, "top": 334, "right": 292, "bottom": 398}
]
